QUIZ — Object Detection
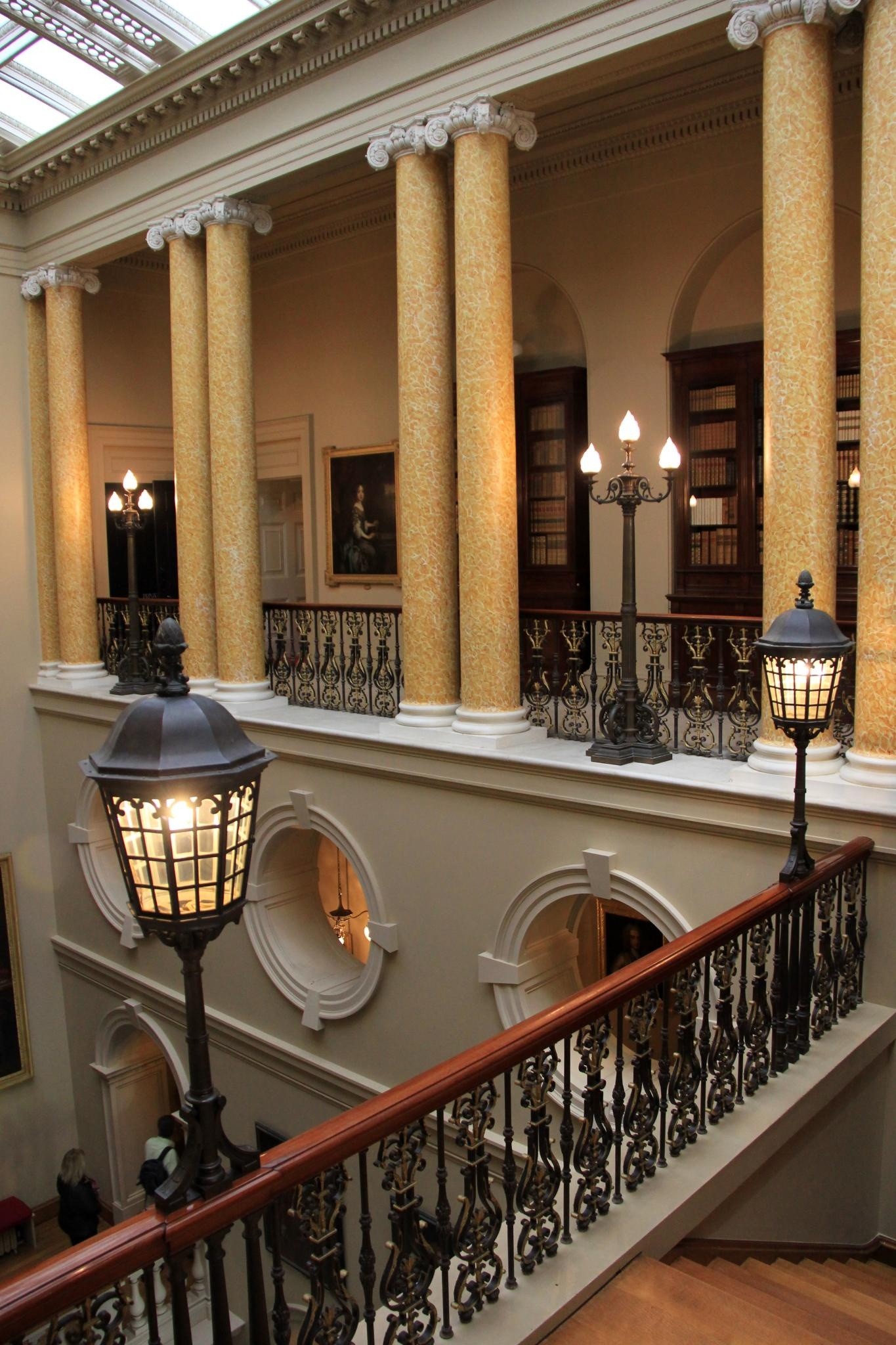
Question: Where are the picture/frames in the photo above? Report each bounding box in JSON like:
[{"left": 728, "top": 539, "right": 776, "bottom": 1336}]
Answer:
[
  {"left": 323, "top": 443, "right": 402, "bottom": 588},
  {"left": 389, "top": 1196, "right": 471, "bottom": 1289},
  {"left": 254, "top": 1117, "right": 347, "bottom": 1301},
  {"left": 596, "top": 898, "right": 670, "bottom": 1013}
]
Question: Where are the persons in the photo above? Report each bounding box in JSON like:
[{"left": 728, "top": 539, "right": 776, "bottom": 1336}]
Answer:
[
  {"left": 143, "top": 1115, "right": 196, "bottom": 1296},
  {"left": 58, "top": 1148, "right": 102, "bottom": 1246}
]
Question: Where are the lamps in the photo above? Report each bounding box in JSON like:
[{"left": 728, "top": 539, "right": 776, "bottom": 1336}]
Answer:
[
  {"left": 76, "top": 620, "right": 276, "bottom": 947},
  {"left": 753, "top": 570, "right": 855, "bottom": 750},
  {"left": 579, "top": 408, "right": 680, "bottom": 506},
  {"left": 109, "top": 470, "right": 155, "bottom": 532},
  {"left": 325, "top": 845, "right": 372, "bottom": 946}
]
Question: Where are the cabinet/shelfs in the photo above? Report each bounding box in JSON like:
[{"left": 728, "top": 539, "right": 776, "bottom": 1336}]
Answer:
[
  {"left": 661, "top": 329, "right": 866, "bottom": 607},
  {"left": 520, "top": 367, "right": 587, "bottom": 609}
]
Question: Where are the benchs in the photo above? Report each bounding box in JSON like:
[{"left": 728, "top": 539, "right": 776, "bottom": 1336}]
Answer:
[{"left": 0, "top": 1196, "right": 37, "bottom": 1254}]
